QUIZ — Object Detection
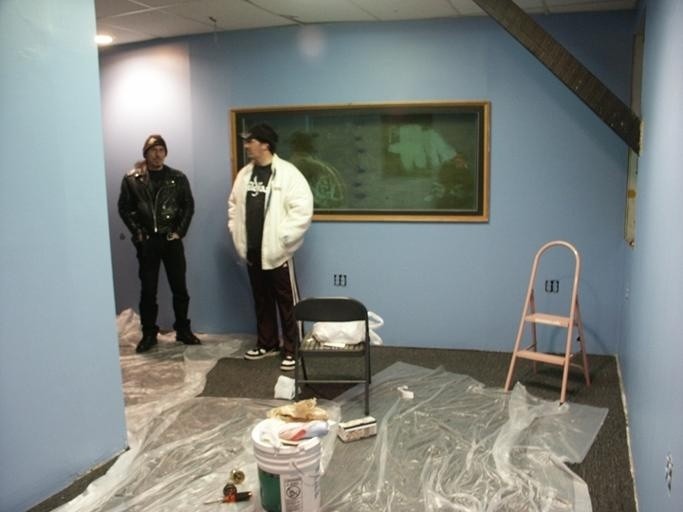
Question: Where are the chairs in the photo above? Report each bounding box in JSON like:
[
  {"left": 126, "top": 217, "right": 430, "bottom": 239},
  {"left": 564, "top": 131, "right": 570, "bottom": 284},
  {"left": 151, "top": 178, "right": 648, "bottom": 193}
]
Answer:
[{"left": 286, "top": 292, "right": 382, "bottom": 417}]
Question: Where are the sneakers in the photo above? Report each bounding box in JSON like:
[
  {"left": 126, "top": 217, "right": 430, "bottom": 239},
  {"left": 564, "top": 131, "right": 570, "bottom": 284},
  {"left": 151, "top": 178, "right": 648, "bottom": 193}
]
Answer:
[
  {"left": 137, "top": 336, "right": 156, "bottom": 352},
  {"left": 177, "top": 333, "right": 199, "bottom": 344},
  {"left": 244, "top": 346, "right": 281, "bottom": 361},
  {"left": 280, "top": 355, "right": 302, "bottom": 371}
]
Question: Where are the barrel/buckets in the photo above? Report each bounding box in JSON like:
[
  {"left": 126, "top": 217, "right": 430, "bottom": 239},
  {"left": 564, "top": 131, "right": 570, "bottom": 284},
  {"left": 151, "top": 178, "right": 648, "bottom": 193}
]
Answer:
[{"left": 251, "top": 416, "right": 321, "bottom": 512}]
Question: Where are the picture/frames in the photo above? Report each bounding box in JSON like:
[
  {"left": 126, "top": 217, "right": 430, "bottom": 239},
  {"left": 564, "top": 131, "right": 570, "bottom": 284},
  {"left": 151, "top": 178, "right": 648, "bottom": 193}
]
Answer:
[{"left": 225, "top": 97, "right": 496, "bottom": 228}]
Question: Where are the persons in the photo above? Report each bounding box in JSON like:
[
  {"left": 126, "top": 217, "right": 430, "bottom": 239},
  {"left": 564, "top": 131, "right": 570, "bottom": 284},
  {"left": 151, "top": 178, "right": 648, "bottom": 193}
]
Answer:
[
  {"left": 226, "top": 125, "right": 314, "bottom": 371},
  {"left": 117, "top": 135, "right": 201, "bottom": 356}
]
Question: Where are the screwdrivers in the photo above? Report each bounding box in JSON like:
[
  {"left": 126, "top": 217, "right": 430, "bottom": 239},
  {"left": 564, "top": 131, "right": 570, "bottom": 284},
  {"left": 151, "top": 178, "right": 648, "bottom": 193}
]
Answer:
[{"left": 203, "top": 491, "right": 253, "bottom": 504}]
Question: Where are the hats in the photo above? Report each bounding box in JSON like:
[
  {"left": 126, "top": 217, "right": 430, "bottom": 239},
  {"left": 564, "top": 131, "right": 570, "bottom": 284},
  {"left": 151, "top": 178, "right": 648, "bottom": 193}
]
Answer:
[
  {"left": 143, "top": 135, "right": 167, "bottom": 158},
  {"left": 239, "top": 124, "right": 278, "bottom": 145}
]
